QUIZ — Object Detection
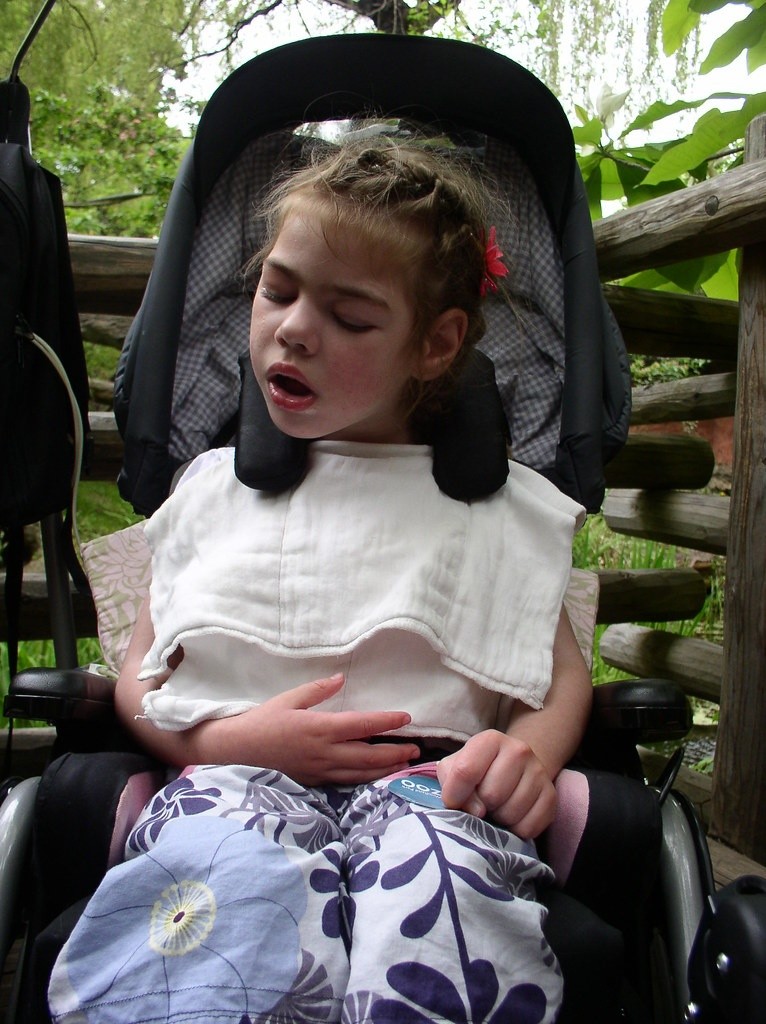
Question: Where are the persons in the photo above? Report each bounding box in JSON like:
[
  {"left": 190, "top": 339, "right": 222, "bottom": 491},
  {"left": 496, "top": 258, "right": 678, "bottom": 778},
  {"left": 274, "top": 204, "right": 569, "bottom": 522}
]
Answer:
[{"left": 45, "top": 127, "right": 596, "bottom": 1024}]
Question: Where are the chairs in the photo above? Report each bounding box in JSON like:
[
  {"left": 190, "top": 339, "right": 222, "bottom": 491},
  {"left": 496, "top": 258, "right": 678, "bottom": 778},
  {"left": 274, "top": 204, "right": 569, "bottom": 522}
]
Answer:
[{"left": 0, "top": 33, "right": 711, "bottom": 1024}]
